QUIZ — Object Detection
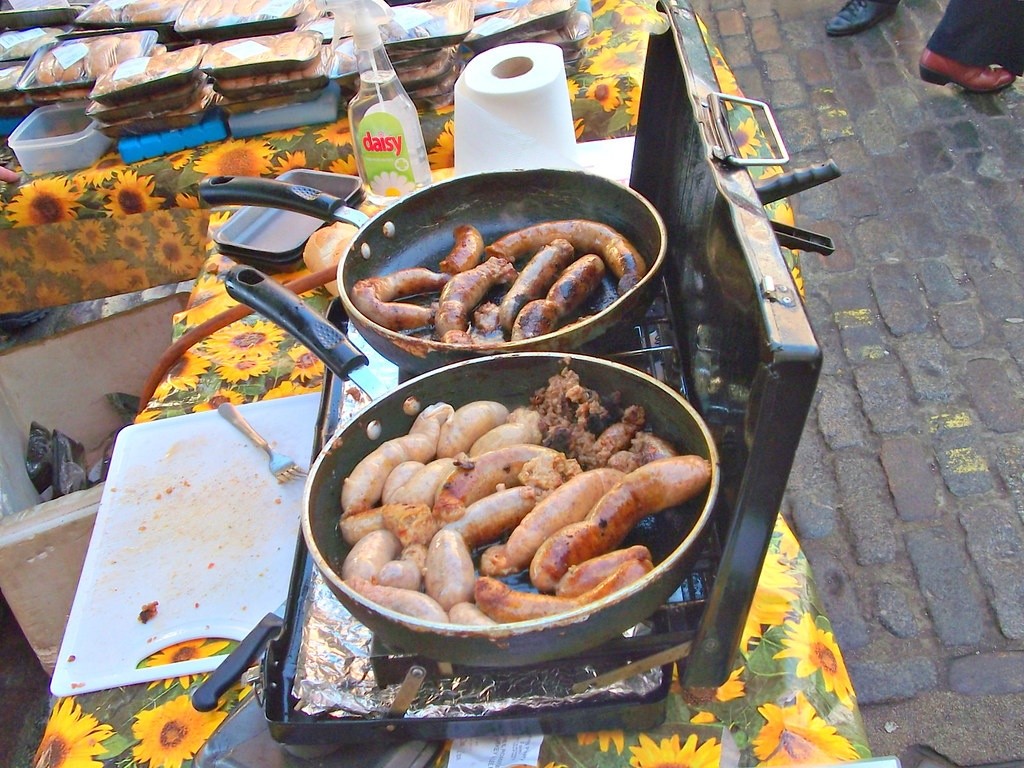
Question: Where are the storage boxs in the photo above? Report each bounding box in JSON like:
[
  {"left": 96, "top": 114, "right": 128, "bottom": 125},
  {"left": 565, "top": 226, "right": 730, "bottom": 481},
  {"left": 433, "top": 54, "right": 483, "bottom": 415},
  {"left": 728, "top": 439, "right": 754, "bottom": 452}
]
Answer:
[{"left": 0, "top": 1, "right": 823, "bottom": 748}]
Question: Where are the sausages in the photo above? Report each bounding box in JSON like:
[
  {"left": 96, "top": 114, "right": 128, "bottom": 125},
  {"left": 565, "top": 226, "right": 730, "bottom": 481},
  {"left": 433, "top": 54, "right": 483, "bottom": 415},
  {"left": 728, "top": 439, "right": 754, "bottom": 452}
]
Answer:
[
  {"left": 338, "top": 400, "right": 714, "bottom": 625},
  {"left": 352, "top": 219, "right": 646, "bottom": 344},
  {"left": 0, "top": 0, "right": 594, "bottom": 130}
]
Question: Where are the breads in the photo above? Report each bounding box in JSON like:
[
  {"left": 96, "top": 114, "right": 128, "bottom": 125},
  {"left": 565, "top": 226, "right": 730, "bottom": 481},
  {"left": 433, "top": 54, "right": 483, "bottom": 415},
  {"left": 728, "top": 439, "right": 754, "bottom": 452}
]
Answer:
[{"left": 303, "top": 221, "right": 365, "bottom": 295}]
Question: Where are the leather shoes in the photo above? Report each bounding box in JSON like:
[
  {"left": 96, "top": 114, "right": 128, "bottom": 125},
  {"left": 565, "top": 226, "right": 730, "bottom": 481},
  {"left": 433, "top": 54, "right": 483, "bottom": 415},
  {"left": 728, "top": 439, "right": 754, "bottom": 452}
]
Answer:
[{"left": 825, "top": 0, "right": 900, "bottom": 35}]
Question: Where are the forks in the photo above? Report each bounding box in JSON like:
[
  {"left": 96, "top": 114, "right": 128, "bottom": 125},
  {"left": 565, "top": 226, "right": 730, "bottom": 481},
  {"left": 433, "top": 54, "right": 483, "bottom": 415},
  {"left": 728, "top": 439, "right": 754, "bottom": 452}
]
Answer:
[{"left": 218, "top": 402, "right": 309, "bottom": 484}]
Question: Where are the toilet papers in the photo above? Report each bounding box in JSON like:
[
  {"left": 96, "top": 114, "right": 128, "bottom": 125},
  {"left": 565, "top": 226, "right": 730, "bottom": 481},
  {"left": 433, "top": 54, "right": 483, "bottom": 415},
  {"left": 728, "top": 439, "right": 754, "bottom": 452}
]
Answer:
[{"left": 453, "top": 38, "right": 582, "bottom": 177}]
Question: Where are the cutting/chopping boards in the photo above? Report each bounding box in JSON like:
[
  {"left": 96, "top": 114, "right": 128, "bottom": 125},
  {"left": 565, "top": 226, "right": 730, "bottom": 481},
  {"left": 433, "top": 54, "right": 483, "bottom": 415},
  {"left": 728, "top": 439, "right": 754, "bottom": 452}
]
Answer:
[{"left": 49, "top": 391, "right": 321, "bottom": 698}]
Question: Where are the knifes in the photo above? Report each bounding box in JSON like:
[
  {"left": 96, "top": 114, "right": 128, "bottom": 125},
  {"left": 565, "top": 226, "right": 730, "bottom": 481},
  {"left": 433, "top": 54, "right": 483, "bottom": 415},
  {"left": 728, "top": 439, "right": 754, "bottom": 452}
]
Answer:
[{"left": 191, "top": 599, "right": 287, "bottom": 712}]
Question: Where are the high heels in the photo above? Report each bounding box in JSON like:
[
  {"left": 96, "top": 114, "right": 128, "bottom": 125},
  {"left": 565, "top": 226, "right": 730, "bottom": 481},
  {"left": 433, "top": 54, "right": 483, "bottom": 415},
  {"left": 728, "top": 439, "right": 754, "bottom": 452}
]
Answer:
[{"left": 919, "top": 46, "right": 1016, "bottom": 93}]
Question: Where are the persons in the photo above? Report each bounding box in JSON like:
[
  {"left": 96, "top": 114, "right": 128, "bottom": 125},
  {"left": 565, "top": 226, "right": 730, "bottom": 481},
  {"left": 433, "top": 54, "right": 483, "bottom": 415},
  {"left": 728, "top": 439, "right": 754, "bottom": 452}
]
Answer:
[
  {"left": 825, "top": 0, "right": 898, "bottom": 36},
  {"left": 919, "top": 0, "right": 1024, "bottom": 92}
]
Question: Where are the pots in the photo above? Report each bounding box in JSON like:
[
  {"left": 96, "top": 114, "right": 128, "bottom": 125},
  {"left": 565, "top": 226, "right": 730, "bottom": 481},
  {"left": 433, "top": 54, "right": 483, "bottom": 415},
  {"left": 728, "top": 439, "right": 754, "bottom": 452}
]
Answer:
[
  {"left": 198, "top": 168, "right": 669, "bottom": 360},
  {"left": 223, "top": 263, "right": 723, "bottom": 667}
]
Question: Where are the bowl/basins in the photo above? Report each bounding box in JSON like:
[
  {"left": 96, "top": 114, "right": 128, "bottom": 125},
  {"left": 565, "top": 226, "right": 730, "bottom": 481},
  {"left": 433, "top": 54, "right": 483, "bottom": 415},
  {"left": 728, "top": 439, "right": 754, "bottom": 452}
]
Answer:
[
  {"left": 212, "top": 167, "right": 367, "bottom": 275},
  {"left": 0, "top": 0, "right": 591, "bottom": 175}
]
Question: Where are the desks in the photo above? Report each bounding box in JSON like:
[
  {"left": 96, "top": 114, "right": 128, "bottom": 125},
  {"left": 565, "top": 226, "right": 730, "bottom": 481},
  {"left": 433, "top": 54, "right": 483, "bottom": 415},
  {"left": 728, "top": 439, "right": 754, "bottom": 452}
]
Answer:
[
  {"left": 0, "top": 0, "right": 805, "bottom": 299},
  {"left": 30, "top": 135, "right": 872, "bottom": 768}
]
